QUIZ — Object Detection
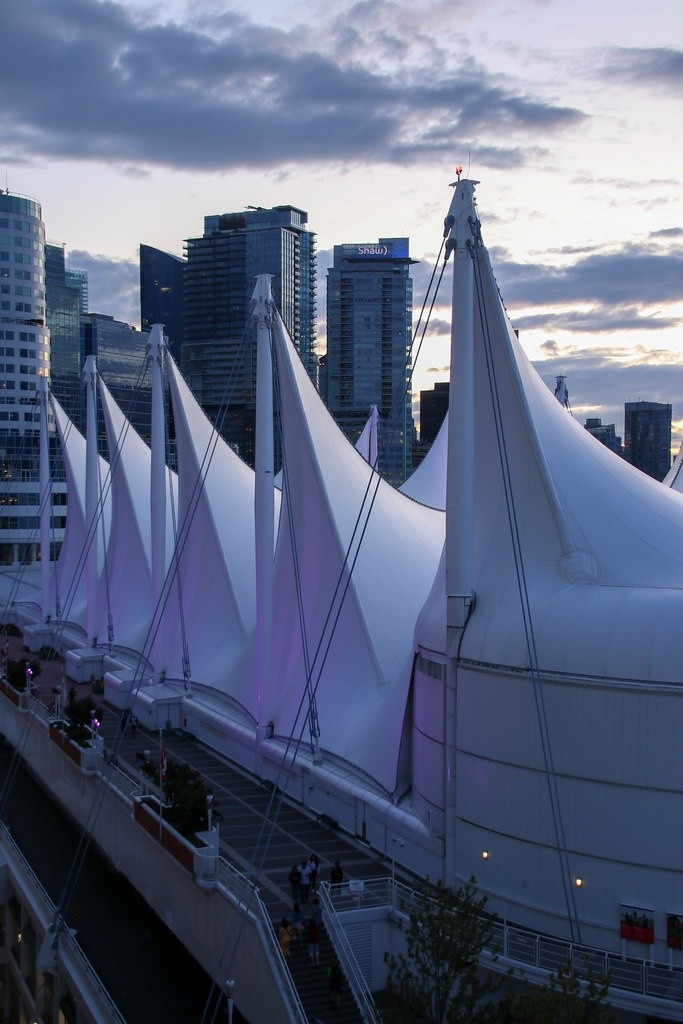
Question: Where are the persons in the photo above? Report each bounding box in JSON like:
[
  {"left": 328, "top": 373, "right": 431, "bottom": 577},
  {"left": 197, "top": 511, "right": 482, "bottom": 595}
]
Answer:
[
  {"left": 144, "top": 748, "right": 150, "bottom": 762},
  {"left": 277, "top": 855, "right": 345, "bottom": 1004},
  {"left": 68, "top": 686, "right": 76, "bottom": 703},
  {"left": 121, "top": 713, "right": 137, "bottom": 737},
  {"left": 165, "top": 784, "right": 173, "bottom": 803}
]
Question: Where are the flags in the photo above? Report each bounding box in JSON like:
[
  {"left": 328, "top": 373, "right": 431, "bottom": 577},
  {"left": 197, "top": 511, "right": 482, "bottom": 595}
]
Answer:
[
  {"left": 3, "top": 631, "right": 9, "bottom": 659},
  {"left": 159, "top": 733, "right": 168, "bottom": 780}
]
